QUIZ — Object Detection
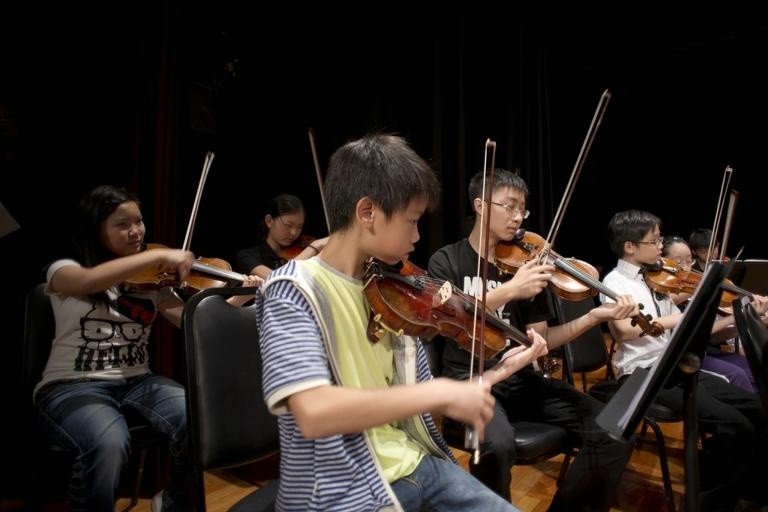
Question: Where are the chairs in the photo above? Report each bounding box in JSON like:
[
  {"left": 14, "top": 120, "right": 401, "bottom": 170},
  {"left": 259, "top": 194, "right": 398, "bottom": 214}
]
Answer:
[{"left": 22, "top": 280, "right": 768, "bottom": 512}]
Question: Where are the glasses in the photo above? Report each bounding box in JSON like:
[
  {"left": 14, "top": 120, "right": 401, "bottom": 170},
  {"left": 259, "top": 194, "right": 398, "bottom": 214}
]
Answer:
[
  {"left": 632, "top": 237, "right": 663, "bottom": 246},
  {"left": 672, "top": 256, "right": 696, "bottom": 266},
  {"left": 484, "top": 200, "right": 530, "bottom": 219}
]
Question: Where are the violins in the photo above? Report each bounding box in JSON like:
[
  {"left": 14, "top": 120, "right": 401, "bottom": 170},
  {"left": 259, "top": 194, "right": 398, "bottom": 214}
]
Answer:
[
  {"left": 280, "top": 234, "right": 316, "bottom": 260},
  {"left": 360, "top": 255, "right": 560, "bottom": 374},
  {"left": 118, "top": 243, "right": 247, "bottom": 294},
  {"left": 637, "top": 258, "right": 754, "bottom": 307},
  {"left": 493, "top": 229, "right": 663, "bottom": 337}
]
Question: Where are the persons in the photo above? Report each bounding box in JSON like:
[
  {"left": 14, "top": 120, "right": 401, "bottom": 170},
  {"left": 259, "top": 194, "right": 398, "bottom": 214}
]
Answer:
[
  {"left": 29, "top": 184, "right": 267, "bottom": 510},
  {"left": 231, "top": 133, "right": 766, "bottom": 511}
]
